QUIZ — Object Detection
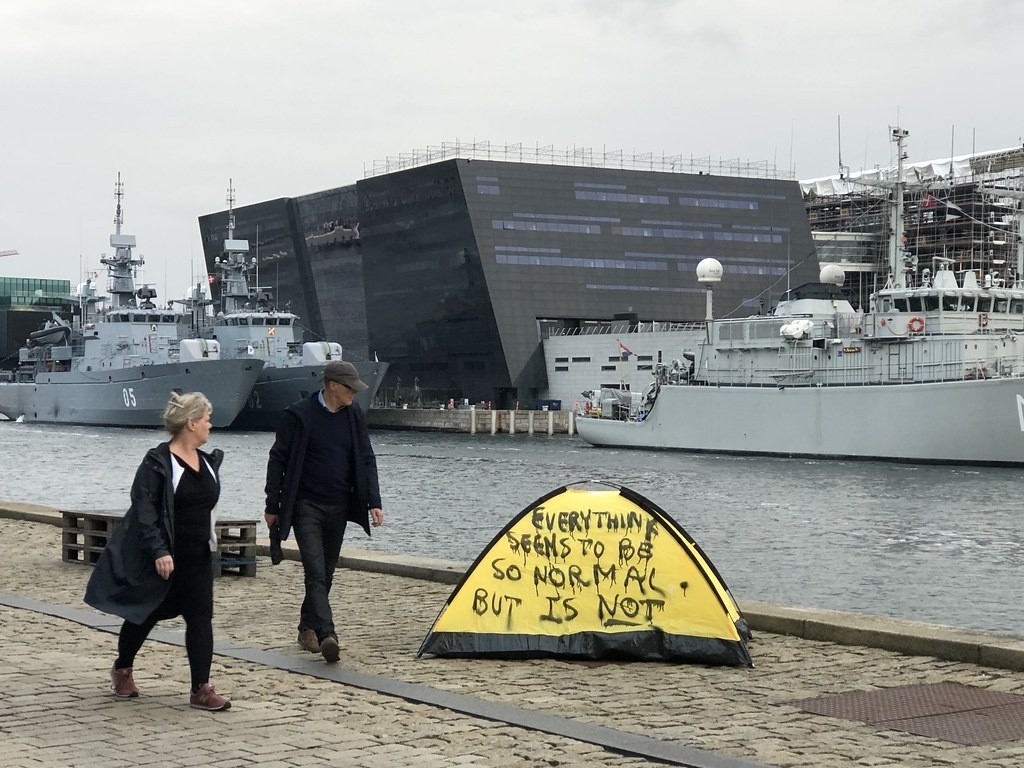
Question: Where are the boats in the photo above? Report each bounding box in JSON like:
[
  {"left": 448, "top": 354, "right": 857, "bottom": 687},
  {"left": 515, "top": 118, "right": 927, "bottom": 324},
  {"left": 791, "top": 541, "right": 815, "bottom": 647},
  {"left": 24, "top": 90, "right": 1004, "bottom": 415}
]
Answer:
[
  {"left": 167, "top": 176, "right": 389, "bottom": 431},
  {"left": 0, "top": 172, "right": 265, "bottom": 428},
  {"left": 575, "top": 117, "right": 1024, "bottom": 468}
]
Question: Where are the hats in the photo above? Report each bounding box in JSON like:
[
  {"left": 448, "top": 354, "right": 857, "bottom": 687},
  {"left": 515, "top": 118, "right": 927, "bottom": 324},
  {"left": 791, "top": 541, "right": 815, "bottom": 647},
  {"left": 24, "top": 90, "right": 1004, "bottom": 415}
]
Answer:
[{"left": 325, "top": 360, "right": 369, "bottom": 391}]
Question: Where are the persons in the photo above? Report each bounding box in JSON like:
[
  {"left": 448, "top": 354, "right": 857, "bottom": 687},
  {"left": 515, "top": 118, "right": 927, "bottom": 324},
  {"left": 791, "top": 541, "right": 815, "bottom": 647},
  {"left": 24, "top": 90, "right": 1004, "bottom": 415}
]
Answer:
[
  {"left": 264, "top": 361, "right": 382, "bottom": 663},
  {"left": 83, "top": 390, "right": 233, "bottom": 711}
]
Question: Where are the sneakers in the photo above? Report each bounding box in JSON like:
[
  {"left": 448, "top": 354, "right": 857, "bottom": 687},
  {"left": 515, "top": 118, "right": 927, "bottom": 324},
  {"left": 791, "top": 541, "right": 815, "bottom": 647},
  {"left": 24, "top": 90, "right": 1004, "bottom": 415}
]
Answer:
[
  {"left": 190, "top": 682, "right": 232, "bottom": 711},
  {"left": 297, "top": 629, "right": 320, "bottom": 653},
  {"left": 110, "top": 659, "right": 139, "bottom": 697},
  {"left": 320, "top": 636, "right": 340, "bottom": 662}
]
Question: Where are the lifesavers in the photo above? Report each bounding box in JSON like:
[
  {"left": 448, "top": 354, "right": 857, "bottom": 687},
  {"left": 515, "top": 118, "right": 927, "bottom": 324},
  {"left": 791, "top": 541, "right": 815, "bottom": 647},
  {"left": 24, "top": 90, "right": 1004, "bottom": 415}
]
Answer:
[
  {"left": 908, "top": 317, "right": 924, "bottom": 332},
  {"left": 978, "top": 313, "right": 988, "bottom": 327}
]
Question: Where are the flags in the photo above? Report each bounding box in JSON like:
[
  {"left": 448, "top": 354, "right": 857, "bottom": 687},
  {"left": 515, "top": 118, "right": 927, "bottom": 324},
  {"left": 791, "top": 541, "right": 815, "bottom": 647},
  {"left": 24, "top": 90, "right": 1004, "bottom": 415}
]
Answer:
[{"left": 620, "top": 343, "right": 633, "bottom": 358}]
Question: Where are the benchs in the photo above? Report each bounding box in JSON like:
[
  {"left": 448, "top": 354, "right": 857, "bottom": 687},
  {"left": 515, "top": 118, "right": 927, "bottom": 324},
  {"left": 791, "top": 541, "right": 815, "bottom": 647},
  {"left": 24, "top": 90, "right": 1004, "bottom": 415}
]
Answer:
[{"left": 58, "top": 507, "right": 260, "bottom": 579}]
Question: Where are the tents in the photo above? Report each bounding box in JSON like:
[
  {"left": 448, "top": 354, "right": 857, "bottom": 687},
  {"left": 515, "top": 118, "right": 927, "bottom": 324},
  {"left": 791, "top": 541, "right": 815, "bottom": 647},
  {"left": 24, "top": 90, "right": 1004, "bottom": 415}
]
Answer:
[{"left": 415, "top": 480, "right": 756, "bottom": 669}]
